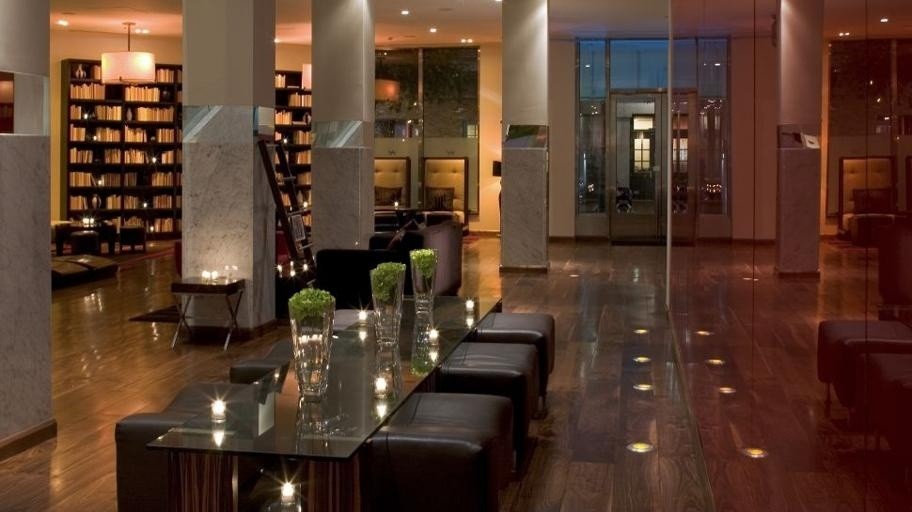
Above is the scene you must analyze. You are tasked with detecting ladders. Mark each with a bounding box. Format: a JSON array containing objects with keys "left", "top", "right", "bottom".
[{"left": 258, "top": 139, "right": 315, "bottom": 272}]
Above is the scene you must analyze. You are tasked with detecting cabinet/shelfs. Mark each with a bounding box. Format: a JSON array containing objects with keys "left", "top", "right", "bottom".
[
  {"left": 60, "top": 58, "right": 183, "bottom": 240},
  {"left": 275, "top": 70, "right": 312, "bottom": 237}
]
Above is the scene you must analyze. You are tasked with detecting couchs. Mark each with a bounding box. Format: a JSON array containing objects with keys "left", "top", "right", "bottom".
[{"left": 316, "top": 219, "right": 465, "bottom": 308}]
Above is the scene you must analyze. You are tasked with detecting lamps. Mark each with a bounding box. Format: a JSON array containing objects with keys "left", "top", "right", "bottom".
[
  {"left": 375, "top": 79, "right": 401, "bottom": 102},
  {"left": 302, "top": 64, "right": 312, "bottom": 92},
  {"left": 101, "top": 22, "right": 156, "bottom": 85}
]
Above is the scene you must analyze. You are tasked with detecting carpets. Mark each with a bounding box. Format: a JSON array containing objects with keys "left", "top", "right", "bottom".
[{"left": 128, "top": 303, "right": 290, "bottom": 326}]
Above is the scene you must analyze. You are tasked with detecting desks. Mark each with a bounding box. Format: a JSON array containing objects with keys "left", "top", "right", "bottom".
[
  {"left": 170, "top": 276, "right": 245, "bottom": 352},
  {"left": 145, "top": 295, "right": 502, "bottom": 512},
  {"left": 55, "top": 222, "right": 117, "bottom": 255}
]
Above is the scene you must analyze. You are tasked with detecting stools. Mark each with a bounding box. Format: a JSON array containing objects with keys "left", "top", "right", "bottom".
[
  {"left": 815, "top": 318, "right": 912, "bottom": 488},
  {"left": 120, "top": 227, "right": 147, "bottom": 253},
  {"left": 70, "top": 230, "right": 100, "bottom": 256}
]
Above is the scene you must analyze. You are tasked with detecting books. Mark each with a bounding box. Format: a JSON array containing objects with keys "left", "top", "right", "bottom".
[
  {"left": 67, "top": 61, "right": 182, "bottom": 234},
  {"left": 274, "top": 72, "right": 312, "bottom": 228}
]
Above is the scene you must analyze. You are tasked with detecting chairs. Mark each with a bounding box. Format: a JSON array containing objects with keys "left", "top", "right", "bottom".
[
  {"left": 839, "top": 155, "right": 898, "bottom": 247},
  {"left": 374, "top": 156, "right": 469, "bottom": 236}
]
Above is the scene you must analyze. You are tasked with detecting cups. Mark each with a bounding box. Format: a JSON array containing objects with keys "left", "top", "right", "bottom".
[
  {"left": 289, "top": 296, "right": 334, "bottom": 396},
  {"left": 370, "top": 268, "right": 405, "bottom": 348},
  {"left": 410, "top": 249, "right": 439, "bottom": 319}
]
